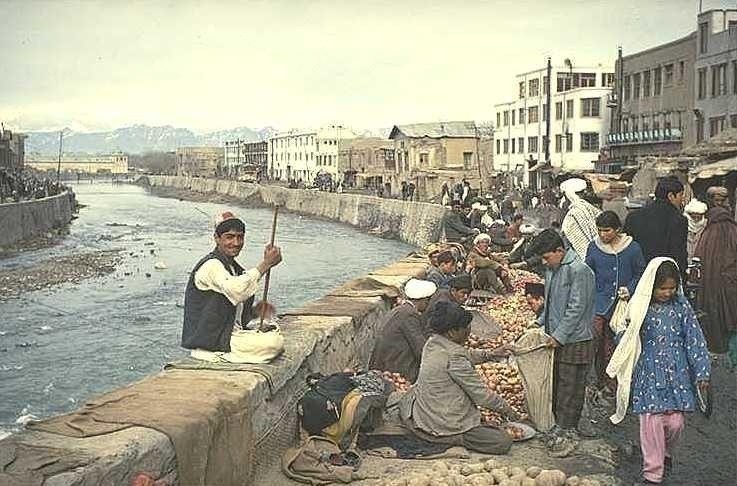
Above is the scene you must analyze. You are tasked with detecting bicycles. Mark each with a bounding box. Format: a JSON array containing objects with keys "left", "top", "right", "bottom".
[{"left": 679, "top": 257, "right": 714, "bottom": 420}]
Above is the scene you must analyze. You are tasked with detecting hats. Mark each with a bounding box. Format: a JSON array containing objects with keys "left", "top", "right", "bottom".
[
  {"left": 472, "top": 201, "right": 537, "bottom": 234},
  {"left": 472, "top": 234, "right": 491, "bottom": 245},
  {"left": 707, "top": 185, "right": 730, "bottom": 200},
  {"left": 212, "top": 207, "right": 241, "bottom": 235},
  {"left": 559, "top": 179, "right": 588, "bottom": 195},
  {"left": 684, "top": 202, "right": 707, "bottom": 214},
  {"left": 403, "top": 277, "right": 437, "bottom": 300}
]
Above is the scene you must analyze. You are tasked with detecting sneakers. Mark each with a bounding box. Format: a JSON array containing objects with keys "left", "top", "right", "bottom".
[{"left": 540, "top": 425, "right": 578, "bottom": 443}]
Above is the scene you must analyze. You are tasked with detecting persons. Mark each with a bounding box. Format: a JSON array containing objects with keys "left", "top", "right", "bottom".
[{"left": 179, "top": 210, "right": 285, "bottom": 364}]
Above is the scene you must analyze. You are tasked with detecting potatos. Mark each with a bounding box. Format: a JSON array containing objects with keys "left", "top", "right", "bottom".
[
  {"left": 342, "top": 247, "right": 560, "bottom": 443},
  {"left": 361, "top": 461, "right": 598, "bottom": 486}
]
